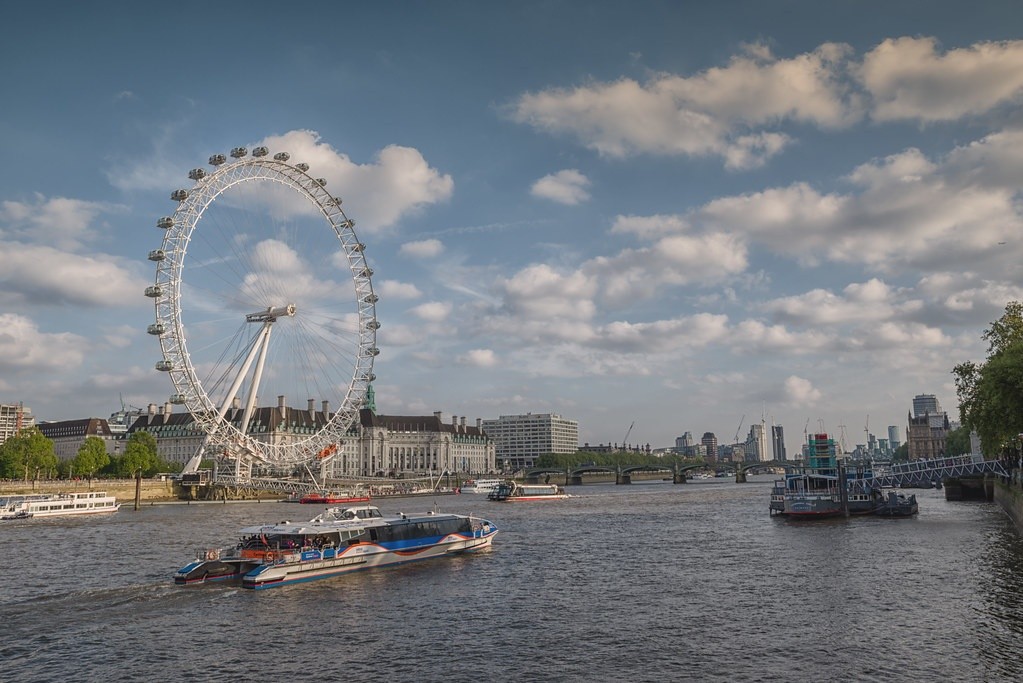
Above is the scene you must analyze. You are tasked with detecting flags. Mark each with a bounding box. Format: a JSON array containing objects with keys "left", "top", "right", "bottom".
[{"left": 260, "top": 532, "right": 267, "bottom": 545}]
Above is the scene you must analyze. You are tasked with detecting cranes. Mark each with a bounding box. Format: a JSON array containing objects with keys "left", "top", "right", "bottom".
[
  {"left": 622, "top": 421, "right": 635, "bottom": 445},
  {"left": 804, "top": 418, "right": 809, "bottom": 442},
  {"left": 864, "top": 414, "right": 869, "bottom": 442},
  {"left": 734, "top": 415, "right": 745, "bottom": 444},
  {"left": 761, "top": 400, "right": 768, "bottom": 424}
]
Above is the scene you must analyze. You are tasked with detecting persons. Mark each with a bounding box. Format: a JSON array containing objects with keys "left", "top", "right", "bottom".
[
  {"left": 239, "top": 534, "right": 268, "bottom": 549},
  {"left": 204, "top": 548, "right": 217, "bottom": 560},
  {"left": 287, "top": 535, "right": 334, "bottom": 552}
]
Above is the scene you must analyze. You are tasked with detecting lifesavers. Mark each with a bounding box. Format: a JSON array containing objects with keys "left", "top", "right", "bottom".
[
  {"left": 209, "top": 550, "right": 217, "bottom": 560},
  {"left": 473, "top": 525, "right": 479, "bottom": 530},
  {"left": 266, "top": 552, "right": 275, "bottom": 560}
]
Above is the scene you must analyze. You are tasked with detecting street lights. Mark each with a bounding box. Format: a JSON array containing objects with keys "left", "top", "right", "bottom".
[
  {"left": 89, "top": 464, "right": 94, "bottom": 489},
  {"left": 32, "top": 465, "right": 38, "bottom": 490}
]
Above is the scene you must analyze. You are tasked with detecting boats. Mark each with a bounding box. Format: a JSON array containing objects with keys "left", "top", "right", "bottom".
[
  {"left": 300, "top": 483, "right": 370, "bottom": 504},
  {"left": 0, "top": 492, "right": 121, "bottom": 519},
  {"left": 173, "top": 506, "right": 499, "bottom": 590},
  {"left": 769, "top": 433, "right": 885, "bottom": 519},
  {"left": 875, "top": 491, "right": 918, "bottom": 516},
  {"left": 506, "top": 484, "right": 572, "bottom": 503},
  {"left": 460, "top": 479, "right": 505, "bottom": 494}
]
